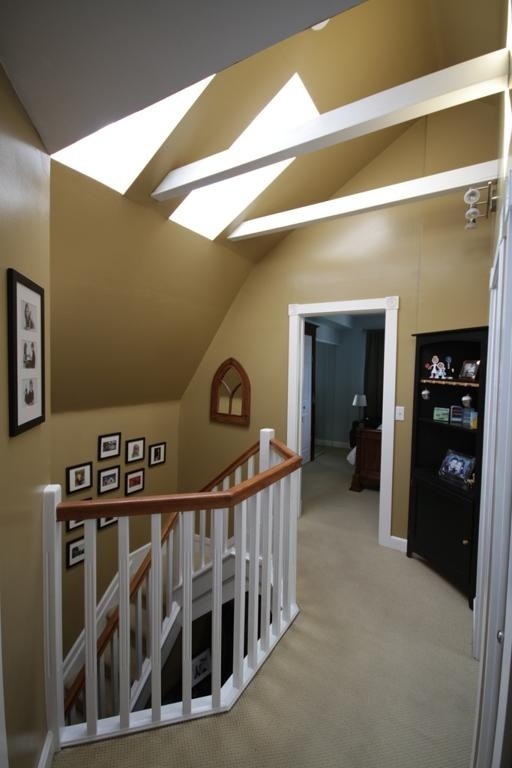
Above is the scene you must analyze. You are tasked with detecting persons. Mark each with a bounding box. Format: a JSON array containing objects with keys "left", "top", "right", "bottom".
[
  {"left": 24, "top": 304, "right": 35, "bottom": 330},
  {"left": 25, "top": 380, "right": 34, "bottom": 404},
  {"left": 446, "top": 457, "right": 465, "bottom": 478},
  {"left": 131, "top": 444, "right": 140, "bottom": 458},
  {"left": 22, "top": 341, "right": 35, "bottom": 368}
]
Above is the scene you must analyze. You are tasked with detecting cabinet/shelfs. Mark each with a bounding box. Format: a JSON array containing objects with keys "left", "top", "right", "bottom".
[{"left": 406, "top": 324, "right": 490, "bottom": 610}]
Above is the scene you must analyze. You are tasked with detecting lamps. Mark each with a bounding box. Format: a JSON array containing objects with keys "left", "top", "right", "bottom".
[
  {"left": 463, "top": 177, "right": 497, "bottom": 232},
  {"left": 352, "top": 393, "right": 368, "bottom": 421}
]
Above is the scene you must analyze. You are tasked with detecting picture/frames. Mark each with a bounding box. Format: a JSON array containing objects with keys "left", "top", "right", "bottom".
[
  {"left": 5, "top": 266, "right": 47, "bottom": 438},
  {"left": 64, "top": 432, "right": 168, "bottom": 569}
]
